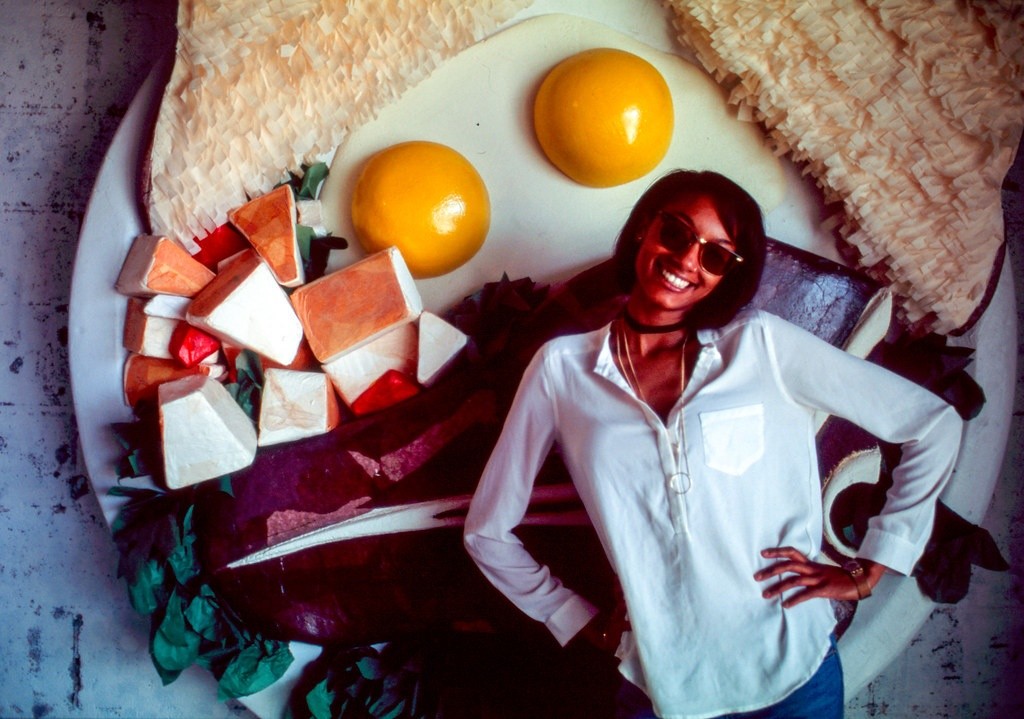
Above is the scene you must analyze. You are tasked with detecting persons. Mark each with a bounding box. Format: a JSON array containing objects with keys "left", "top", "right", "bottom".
[{"left": 463, "top": 171, "right": 964, "bottom": 719}]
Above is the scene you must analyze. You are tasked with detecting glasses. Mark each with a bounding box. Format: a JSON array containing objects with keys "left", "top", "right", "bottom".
[{"left": 653, "top": 209, "right": 745, "bottom": 277}]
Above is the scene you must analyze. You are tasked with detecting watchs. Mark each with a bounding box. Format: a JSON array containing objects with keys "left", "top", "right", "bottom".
[{"left": 843, "top": 561, "right": 872, "bottom": 600}]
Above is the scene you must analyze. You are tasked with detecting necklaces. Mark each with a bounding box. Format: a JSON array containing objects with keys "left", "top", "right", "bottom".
[{"left": 608, "top": 306, "right": 694, "bottom": 493}]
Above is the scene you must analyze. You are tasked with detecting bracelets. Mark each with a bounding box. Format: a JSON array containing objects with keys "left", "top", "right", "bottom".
[{"left": 601, "top": 617, "right": 611, "bottom": 656}]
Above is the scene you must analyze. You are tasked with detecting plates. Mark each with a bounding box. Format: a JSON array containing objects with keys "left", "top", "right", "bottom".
[{"left": 67, "top": 2, "right": 1018, "bottom": 719}]
